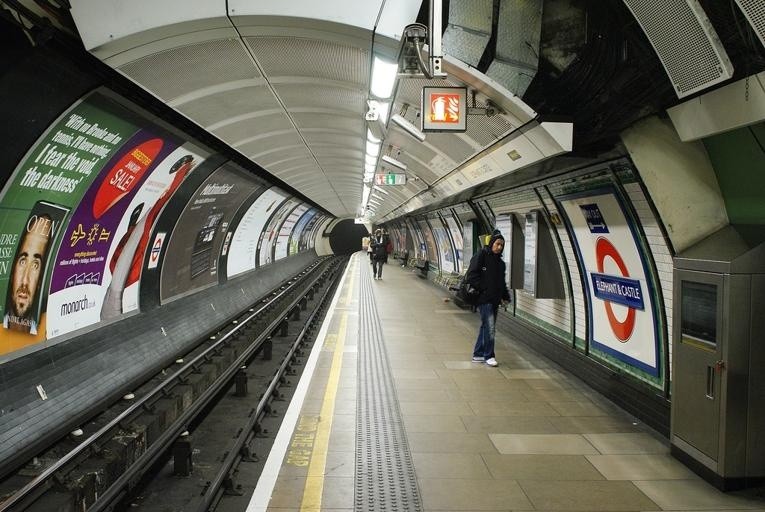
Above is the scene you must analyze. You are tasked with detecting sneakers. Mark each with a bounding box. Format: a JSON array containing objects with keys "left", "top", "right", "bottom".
[
  {"left": 486, "top": 358, "right": 498, "bottom": 366},
  {"left": 472, "top": 357, "right": 484, "bottom": 363}
]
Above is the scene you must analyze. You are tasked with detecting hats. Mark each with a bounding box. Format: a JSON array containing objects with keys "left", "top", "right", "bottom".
[{"left": 489, "top": 230, "right": 504, "bottom": 253}]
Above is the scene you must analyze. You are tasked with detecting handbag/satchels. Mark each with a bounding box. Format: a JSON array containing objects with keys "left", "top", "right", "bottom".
[{"left": 453, "top": 267, "right": 487, "bottom": 310}]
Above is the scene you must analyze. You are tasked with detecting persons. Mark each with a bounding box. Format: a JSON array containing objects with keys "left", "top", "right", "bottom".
[
  {"left": 11, "top": 214, "right": 52, "bottom": 317},
  {"left": 366, "top": 233, "right": 393, "bottom": 264},
  {"left": 460, "top": 229, "right": 512, "bottom": 366},
  {"left": 373, "top": 229, "right": 385, "bottom": 281}
]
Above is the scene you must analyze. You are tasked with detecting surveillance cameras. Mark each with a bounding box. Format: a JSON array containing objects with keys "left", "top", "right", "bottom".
[
  {"left": 366, "top": 108, "right": 388, "bottom": 140},
  {"left": 397, "top": 22, "right": 432, "bottom": 79},
  {"left": 362, "top": 177, "right": 372, "bottom": 188}
]
